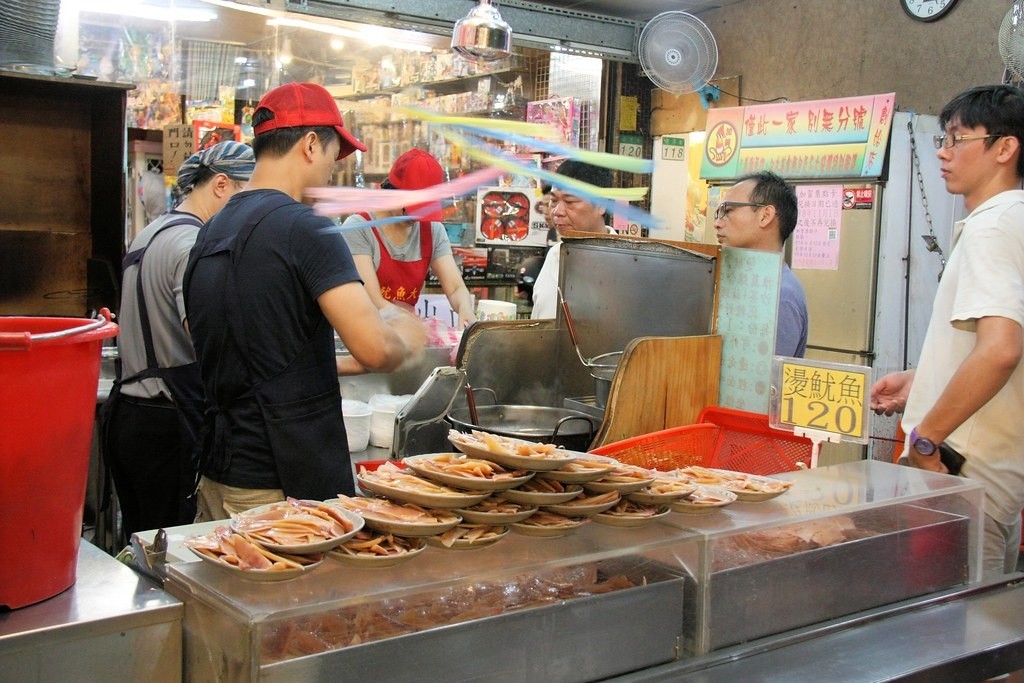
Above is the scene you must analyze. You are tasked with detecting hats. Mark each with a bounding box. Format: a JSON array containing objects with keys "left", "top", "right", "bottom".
[
  {"left": 253, "top": 82, "right": 367, "bottom": 160},
  {"left": 390, "top": 148, "right": 447, "bottom": 222}
]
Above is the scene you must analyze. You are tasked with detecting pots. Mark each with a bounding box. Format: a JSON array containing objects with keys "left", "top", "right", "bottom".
[{"left": 447, "top": 387, "right": 601, "bottom": 452}]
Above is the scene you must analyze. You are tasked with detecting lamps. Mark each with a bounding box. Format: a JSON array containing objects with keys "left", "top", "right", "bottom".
[{"left": 451, "top": 1, "right": 513, "bottom": 62}]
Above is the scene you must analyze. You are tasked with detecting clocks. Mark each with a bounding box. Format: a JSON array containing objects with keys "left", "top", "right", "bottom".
[{"left": 900, "top": 0, "right": 958, "bottom": 22}]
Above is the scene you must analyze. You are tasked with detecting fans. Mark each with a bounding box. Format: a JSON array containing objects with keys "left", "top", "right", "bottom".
[
  {"left": 998, "top": 0, "right": 1024, "bottom": 92},
  {"left": 638, "top": 12, "right": 721, "bottom": 109}
]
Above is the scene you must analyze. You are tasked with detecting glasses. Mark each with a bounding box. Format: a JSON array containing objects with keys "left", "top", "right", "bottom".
[
  {"left": 712, "top": 201, "right": 779, "bottom": 221},
  {"left": 933, "top": 131, "right": 1005, "bottom": 150}
]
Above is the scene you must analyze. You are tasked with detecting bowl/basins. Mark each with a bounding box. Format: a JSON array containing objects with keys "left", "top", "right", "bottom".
[
  {"left": 476, "top": 299, "right": 517, "bottom": 322},
  {"left": 344, "top": 394, "right": 395, "bottom": 453}
]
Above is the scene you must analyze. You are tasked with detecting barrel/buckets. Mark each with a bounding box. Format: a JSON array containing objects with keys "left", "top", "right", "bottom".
[
  {"left": 0, "top": 306, "right": 119, "bottom": 610},
  {"left": 590, "top": 372, "right": 613, "bottom": 409},
  {"left": 442, "top": 222, "right": 467, "bottom": 243}
]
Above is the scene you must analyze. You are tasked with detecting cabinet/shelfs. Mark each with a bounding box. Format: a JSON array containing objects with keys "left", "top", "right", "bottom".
[{"left": 332, "top": 67, "right": 529, "bottom": 185}]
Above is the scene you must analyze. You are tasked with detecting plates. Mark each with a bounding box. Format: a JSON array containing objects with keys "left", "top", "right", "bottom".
[{"left": 187, "top": 433, "right": 790, "bottom": 584}]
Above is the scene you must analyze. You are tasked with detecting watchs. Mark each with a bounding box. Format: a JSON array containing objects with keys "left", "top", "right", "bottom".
[{"left": 907, "top": 426, "right": 941, "bottom": 456}]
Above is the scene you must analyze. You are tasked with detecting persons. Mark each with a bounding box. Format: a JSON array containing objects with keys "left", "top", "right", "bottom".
[
  {"left": 339, "top": 149, "right": 481, "bottom": 349},
  {"left": 714, "top": 172, "right": 809, "bottom": 359},
  {"left": 530, "top": 156, "right": 621, "bottom": 321},
  {"left": 182, "top": 85, "right": 425, "bottom": 525},
  {"left": 870, "top": 85, "right": 1024, "bottom": 583},
  {"left": 113, "top": 140, "right": 257, "bottom": 527}
]
